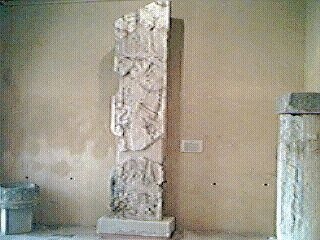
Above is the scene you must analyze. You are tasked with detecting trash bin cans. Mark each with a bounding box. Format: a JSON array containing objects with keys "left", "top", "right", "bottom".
[{"left": 1, "top": 182, "right": 40, "bottom": 235}]
